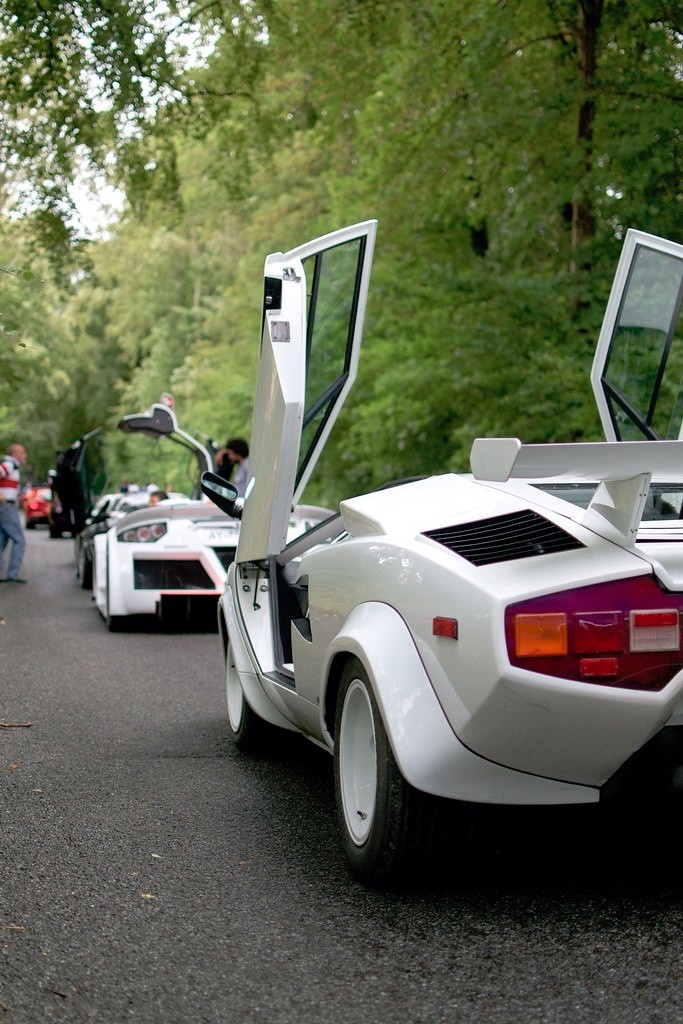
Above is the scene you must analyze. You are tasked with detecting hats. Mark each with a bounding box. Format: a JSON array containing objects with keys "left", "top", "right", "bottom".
[{"left": 226, "top": 439, "right": 249, "bottom": 458}]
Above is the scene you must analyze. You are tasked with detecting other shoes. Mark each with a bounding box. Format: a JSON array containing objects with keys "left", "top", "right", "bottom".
[{"left": 1, "top": 577, "right": 29, "bottom": 584}]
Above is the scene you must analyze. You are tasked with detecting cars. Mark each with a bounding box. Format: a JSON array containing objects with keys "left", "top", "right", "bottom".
[
  {"left": 75, "top": 485, "right": 190, "bottom": 587},
  {"left": 21, "top": 481, "right": 52, "bottom": 529}
]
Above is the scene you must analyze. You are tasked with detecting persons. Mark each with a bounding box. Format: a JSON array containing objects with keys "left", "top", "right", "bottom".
[
  {"left": 0, "top": 443, "right": 28, "bottom": 584},
  {"left": 148, "top": 493, "right": 168, "bottom": 506},
  {"left": 115, "top": 478, "right": 172, "bottom": 493},
  {"left": 214, "top": 438, "right": 249, "bottom": 497}
]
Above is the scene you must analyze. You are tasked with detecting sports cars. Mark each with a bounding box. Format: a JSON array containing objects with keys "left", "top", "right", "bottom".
[
  {"left": 92, "top": 399, "right": 339, "bottom": 632},
  {"left": 216, "top": 218, "right": 681, "bottom": 888}
]
